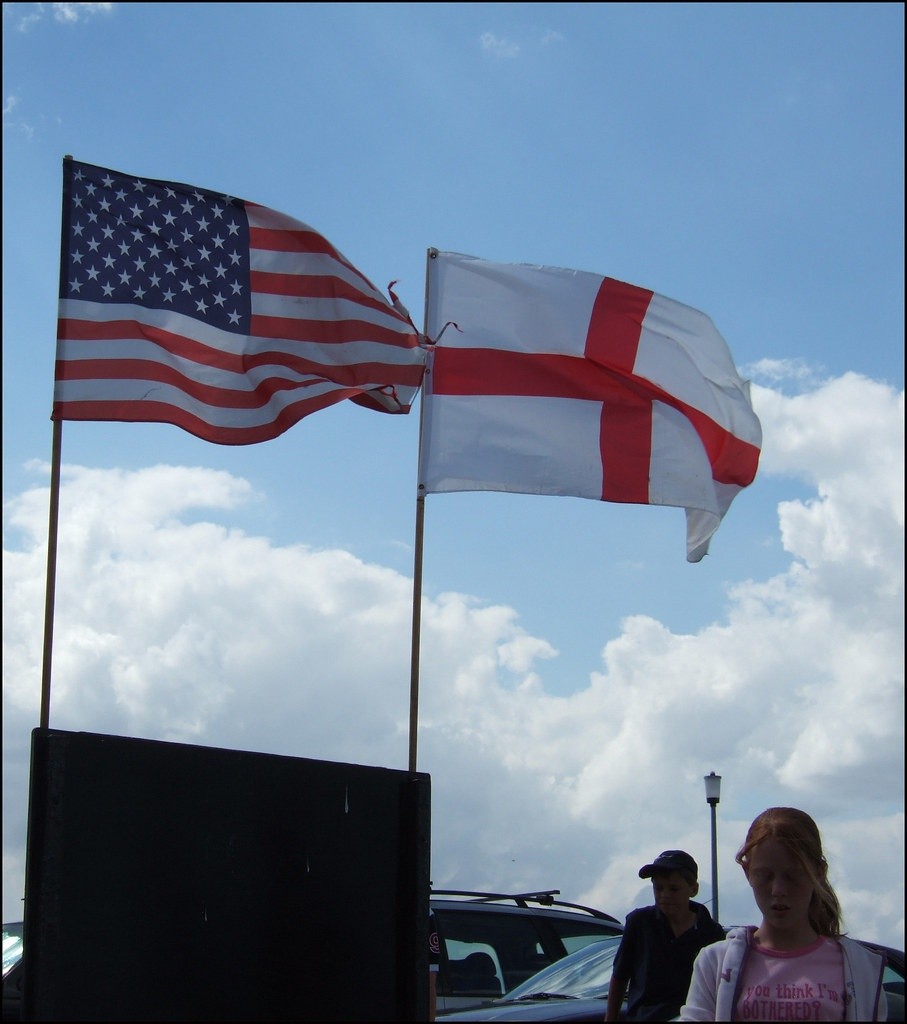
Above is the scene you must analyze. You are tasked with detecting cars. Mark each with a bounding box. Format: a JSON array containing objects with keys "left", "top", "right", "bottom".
[{"left": 432, "top": 884, "right": 906, "bottom": 1024}]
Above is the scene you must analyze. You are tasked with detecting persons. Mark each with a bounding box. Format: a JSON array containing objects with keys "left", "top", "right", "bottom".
[
  {"left": 667, "top": 808, "right": 890, "bottom": 1022},
  {"left": 427, "top": 907, "right": 444, "bottom": 1022},
  {"left": 606, "top": 849, "right": 727, "bottom": 1021}
]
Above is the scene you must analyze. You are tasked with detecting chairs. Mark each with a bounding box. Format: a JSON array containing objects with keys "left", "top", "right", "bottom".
[{"left": 448, "top": 952, "right": 501, "bottom": 990}]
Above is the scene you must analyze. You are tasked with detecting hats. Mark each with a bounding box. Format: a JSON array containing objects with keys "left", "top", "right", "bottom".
[{"left": 639, "top": 851, "right": 698, "bottom": 879}]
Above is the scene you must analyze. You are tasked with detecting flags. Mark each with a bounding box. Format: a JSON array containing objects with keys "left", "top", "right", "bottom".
[
  {"left": 417, "top": 247, "right": 762, "bottom": 564},
  {"left": 52, "top": 157, "right": 433, "bottom": 448}
]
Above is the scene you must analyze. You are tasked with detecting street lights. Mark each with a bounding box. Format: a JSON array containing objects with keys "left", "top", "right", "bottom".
[{"left": 702, "top": 771, "right": 723, "bottom": 923}]
